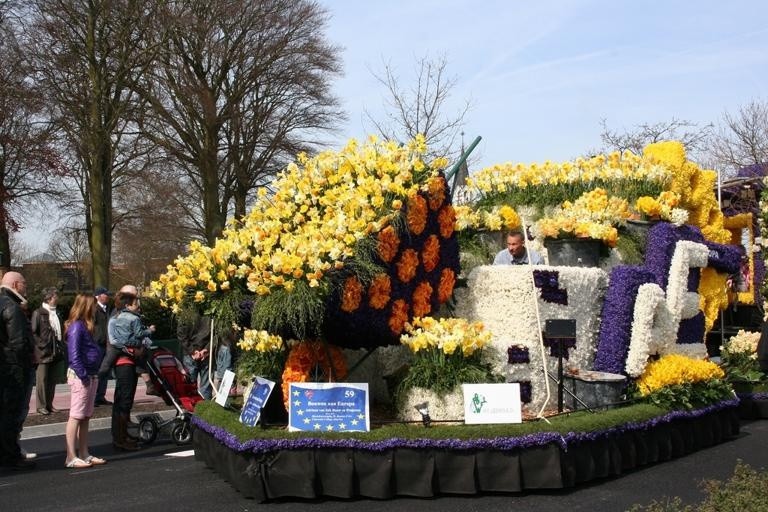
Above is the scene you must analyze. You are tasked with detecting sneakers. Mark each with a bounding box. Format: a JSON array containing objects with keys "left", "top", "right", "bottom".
[
  {"left": 93, "top": 399, "right": 112, "bottom": 407},
  {"left": 126, "top": 422, "right": 140, "bottom": 428},
  {"left": 112, "top": 441, "right": 141, "bottom": 452},
  {"left": 37, "top": 408, "right": 49, "bottom": 414},
  {"left": 125, "top": 435, "right": 145, "bottom": 443},
  {"left": 0, "top": 461, "right": 36, "bottom": 472},
  {"left": 46, "top": 407, "right": 59, "bottom": 413}
]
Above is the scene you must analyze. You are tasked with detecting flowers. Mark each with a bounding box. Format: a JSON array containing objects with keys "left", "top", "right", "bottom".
[
  {"left": 536, "top": 211, "right": 619, "bottom": 246},
  {"left": 453, "top": 204, "right": 521, "bottom": 233}
]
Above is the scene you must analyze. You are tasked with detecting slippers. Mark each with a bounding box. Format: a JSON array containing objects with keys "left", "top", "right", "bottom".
[
  {"left": 65, "top": 457, "right": 92, "bottom": 468},
  {"left": 84, "top": 455, "right": 106, "bottom": 464}
]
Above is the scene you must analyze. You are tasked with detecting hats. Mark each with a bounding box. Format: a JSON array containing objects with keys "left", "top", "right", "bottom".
[{"left": 94, "top": 287, "right": 112, "bottom": 296}]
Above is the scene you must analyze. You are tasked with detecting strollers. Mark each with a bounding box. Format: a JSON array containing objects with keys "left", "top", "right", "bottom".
[{"left": 137, "top": 340, "right": 205, "bottom": 445}]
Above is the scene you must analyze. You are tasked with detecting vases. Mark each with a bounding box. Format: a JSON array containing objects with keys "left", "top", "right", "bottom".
[
  {"left": 477, "top": 229, "right": 505, "bottom": 254},
  {"left": 541, "top": 239, "right": 601, "bottom": 267}
]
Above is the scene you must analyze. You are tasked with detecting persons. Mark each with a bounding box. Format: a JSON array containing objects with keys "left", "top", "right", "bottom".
[
  {"left": 64, "top": 294, "right": 107, "bottom": 468},
  {"left": 107, "top": 285, "right": 144, "bottom": 451},
  {"left": 17, "top": 294, "right": 37, "bottom": 459},
  {"left": 0, "top": 271, "right": 37, "bottom": 471},
  {"left": 217, "top": 344, "right": 237, "bottom": 395},
  {"left": 90, "top": 287, "right": 113, "bottom": 407},
  {"left": 178, "top": 313, "right": 211, "bottom": 401},
  {"left": 31, "top": 286, "right": 65, "bottom": 414},
  {"left": 493, "top": 232, "right": 544, "bottom": 265},
  {"left": 95, "top": 293, "right": 161, "bottom": 396}
]
